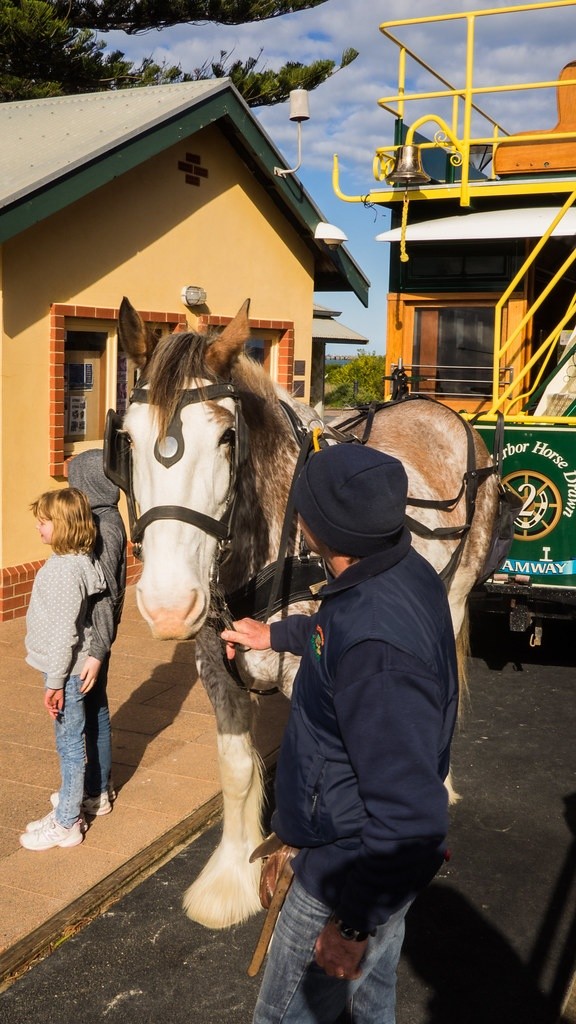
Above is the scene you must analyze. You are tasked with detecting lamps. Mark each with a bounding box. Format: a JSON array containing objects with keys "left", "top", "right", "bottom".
[
  {"left": 181, "top": 285, "right": 208, "bottom": 307},
  {"left": 273, "top": 85, "right": 310, "bottom": 179}
]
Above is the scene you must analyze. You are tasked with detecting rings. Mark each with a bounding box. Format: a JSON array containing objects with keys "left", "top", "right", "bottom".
[{"left": 337, "top": 974, "right": 344, "bottom": 977}]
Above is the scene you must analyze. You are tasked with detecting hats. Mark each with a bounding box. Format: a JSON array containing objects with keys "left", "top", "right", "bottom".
[{"left": 291, "top": 443, "right": 409, "bottom": 558}]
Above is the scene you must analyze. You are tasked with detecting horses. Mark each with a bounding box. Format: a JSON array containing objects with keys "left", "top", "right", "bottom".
[{"left": 113, "top": 292, "right": 518, "bottom": 927}]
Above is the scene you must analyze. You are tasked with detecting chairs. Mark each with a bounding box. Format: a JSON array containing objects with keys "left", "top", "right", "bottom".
[{"left": 494, "top": 60, "right": 576, "bottom": 176}]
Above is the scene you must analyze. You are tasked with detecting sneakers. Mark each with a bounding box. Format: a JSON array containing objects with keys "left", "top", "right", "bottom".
[
  {"left": 50, "top": 781, "right": 116, "bottom": 816},
  {"left": 25, "top": 808, "right": 89, "bottom": 834},
  {"left": 19, "top": 819, "right": 83, "bottom": 851}
]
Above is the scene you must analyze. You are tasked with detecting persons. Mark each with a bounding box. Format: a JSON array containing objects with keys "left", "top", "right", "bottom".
[
  {"left": 51, "top": 450, "right": 128, "bottom": 816},
  {"left": 19, "top": 487, "right": 107, "bottom": 851},
  {"left": 220, "top": 444, "right": 458, "bottom": 1024}
]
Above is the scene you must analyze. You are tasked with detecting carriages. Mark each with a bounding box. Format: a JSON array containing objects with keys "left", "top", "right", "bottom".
[{"left": 102, "top": 0, "right": 576, "bottom": 931}]
